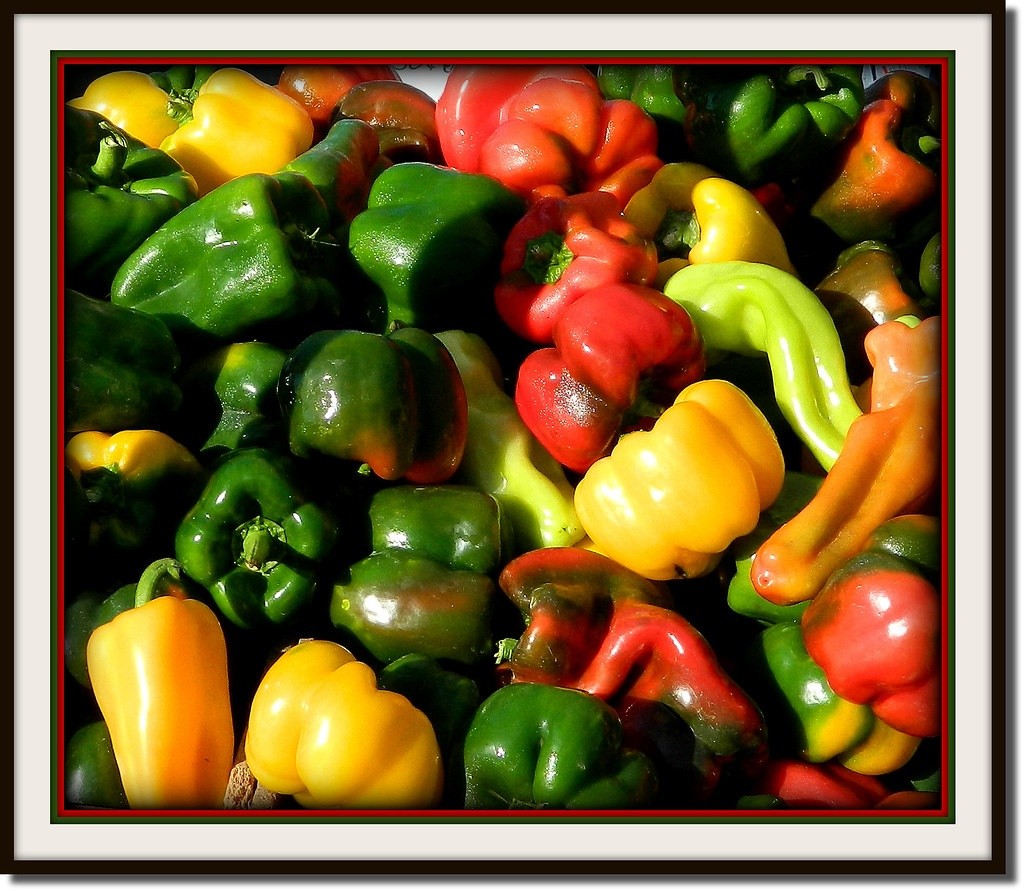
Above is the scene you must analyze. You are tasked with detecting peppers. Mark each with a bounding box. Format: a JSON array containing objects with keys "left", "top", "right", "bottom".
[{"left": 65, "top": 66, "right": 943, "bottom": 809}]
